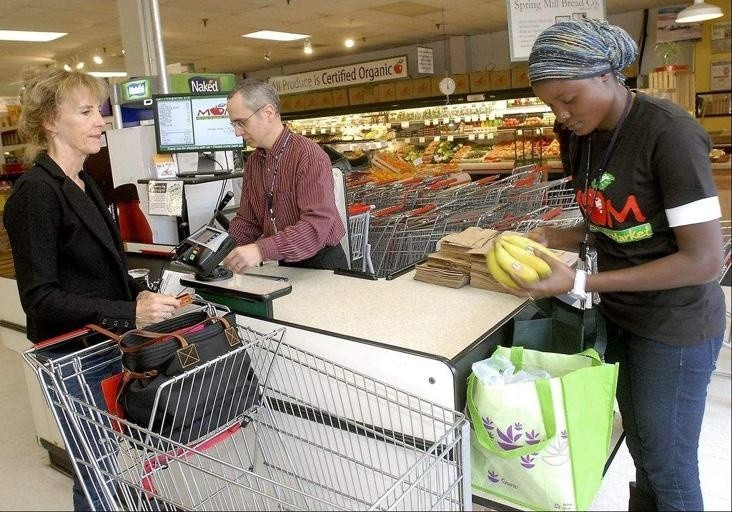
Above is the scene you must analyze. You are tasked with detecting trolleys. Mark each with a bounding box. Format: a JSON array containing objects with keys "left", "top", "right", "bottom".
[
  {"left": 345, "top": 160, "right": 582, "bottom": 280},
  {"left": 16, "top": 294, "right": 475, "bottom": 512},
  {"left": 715, "top": 218, "right": 732, "bottom": 350}
]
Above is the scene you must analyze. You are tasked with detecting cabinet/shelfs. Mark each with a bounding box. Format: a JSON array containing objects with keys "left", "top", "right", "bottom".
[
  {"left": 280, "top": 97, "right": 570, "bottom": 172},
  {"left": 0, "top": 112, "right": 49, "bottom": 327}
]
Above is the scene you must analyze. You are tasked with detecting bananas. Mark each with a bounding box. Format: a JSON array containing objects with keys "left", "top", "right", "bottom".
[{"left": 487, "top": 234, "right": 560, "bottom": 291}]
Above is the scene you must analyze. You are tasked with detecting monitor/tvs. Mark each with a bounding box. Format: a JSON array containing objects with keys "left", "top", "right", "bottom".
[{"left": 152, "top": 91, "right": 246, "bottom": 177}]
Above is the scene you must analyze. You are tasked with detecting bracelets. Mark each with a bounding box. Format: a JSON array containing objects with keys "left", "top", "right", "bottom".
[{"left": 566, "top": 266, "right": 596, "bottom": 313}]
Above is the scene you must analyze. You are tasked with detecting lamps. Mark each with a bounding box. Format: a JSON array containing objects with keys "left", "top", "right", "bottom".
[
  {"left": 304, "top": 39, "right": 314, "bottom": 54},
  {"left": 675, "top": 0, "right": 724, "bottom": 23},
  {"left": 264, "top": 50, "right": 272, "bottom": 61}
]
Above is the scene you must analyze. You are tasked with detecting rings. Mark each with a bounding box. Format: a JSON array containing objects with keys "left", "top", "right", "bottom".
[
  {"left": 164, "top": 313, "right": 172, "bottom": 319},
  {"left": 525, "top": 290, "right": 537, "bottom": 303}
]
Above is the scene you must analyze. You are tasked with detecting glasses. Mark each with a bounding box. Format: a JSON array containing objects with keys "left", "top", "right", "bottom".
[{"left": 228, "top": 107, "right": 263, "bottom": 130}]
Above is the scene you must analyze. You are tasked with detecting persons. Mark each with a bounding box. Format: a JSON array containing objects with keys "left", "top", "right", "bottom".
[
  {"left": 501, "top": 16, "right": 729, "bottom": 512},
  {"left": 218, "top": 74, "right": 353, "bottom": 277},
  {"left": 0, "top": 61, "right": 182, "bottom": 512}
]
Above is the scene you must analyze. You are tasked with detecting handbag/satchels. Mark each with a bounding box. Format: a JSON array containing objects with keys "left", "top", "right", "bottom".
[
  {"left": 462, "top": 345, "right": 620, "bottom": 512},
  {"left": 118, "top": 311, "right": 259, "bottom": 454}
]
